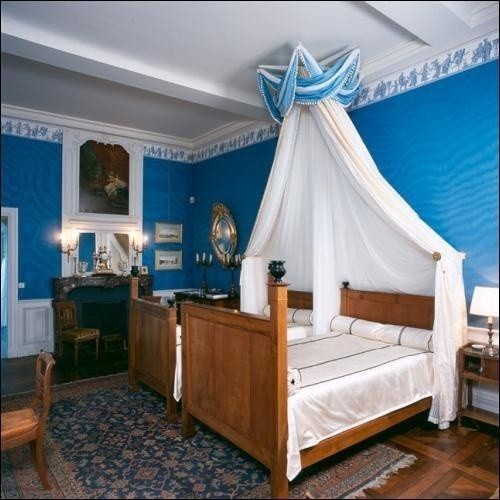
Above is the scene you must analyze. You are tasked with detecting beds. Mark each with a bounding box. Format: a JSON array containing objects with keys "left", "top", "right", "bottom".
[
  {"left": 181, "top": 260, "right": 453, "bottom": 500},
  {"left": 126, "top": 264, "right": 313, "bottom": 427}
]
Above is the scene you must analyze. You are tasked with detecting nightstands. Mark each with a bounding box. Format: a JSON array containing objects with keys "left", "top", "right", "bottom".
[{"left": 456, "top": 341, "right": 500, "bottom": 434}]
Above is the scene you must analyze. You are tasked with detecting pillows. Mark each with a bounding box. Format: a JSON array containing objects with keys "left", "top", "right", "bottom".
[
  {"left": 330, "top": 314, "right": 434, "bottom": 351},
  {"left": 264, "top": 304, "right": 313, "bottom": 324}
]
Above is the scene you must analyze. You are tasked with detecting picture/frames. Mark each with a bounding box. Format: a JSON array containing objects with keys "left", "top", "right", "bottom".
[
  {"left": 155, "top": 222, "right": 183, "bottom": 244},
  {"left": 155, "top": 249, "right": 182, "bottom": 271},
  {"left": 68, "top": 131, "right": 138, "bottom": 225},
  {"left": 209, "top": 202, "right": 237, "bottom": 264}
]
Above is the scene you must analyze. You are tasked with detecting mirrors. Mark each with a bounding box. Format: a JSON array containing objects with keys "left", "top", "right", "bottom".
[{"left": 76, "top": 230, "right": 134, "bottom": 276}]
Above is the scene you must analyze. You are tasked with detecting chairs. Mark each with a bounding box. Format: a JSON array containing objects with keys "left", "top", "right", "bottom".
[
  {"left": 0, "top": 350, "right": 58, "bottom": 491},
  {"left": 53, "top": 299, "right": 103, "bottom": 368}
]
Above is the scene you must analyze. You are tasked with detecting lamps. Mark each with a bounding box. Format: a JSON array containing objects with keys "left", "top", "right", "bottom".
[{"left": 469, "top": 285, "right": 499, "bottom": 353}]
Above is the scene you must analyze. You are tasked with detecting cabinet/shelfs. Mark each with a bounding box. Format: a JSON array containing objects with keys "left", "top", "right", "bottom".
[{"left": 173, "top": 289, "right": 239, "bottom": 326}]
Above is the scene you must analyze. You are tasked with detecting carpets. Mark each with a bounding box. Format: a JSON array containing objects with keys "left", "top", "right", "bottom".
[{"left": 0, "top": 371, "right": 422, "bottom": 499}]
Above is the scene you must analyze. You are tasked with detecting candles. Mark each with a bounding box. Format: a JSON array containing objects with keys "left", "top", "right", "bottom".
[{"left": 195, "top": 252, "right": 241, "bottom": 265}]
